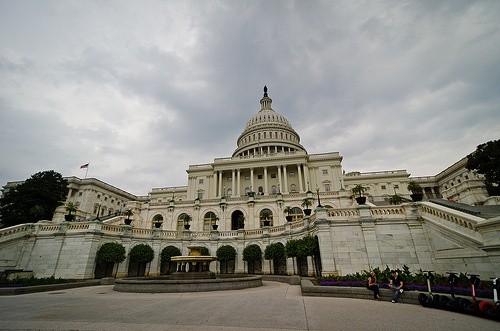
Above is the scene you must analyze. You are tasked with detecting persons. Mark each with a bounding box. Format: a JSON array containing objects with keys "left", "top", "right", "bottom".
[
  {"left": 389, "top": 270, "right": 403, "bottom": 302},
  {"left": 366, "top": 271, "right": 381, "bottom": 300}
]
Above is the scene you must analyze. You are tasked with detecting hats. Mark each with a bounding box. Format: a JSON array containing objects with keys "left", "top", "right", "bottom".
[{"left": 390, "top": 270, "right": 396, "bottom": 273}]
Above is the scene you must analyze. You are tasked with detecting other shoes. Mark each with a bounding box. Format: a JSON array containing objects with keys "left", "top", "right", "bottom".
[
  {"left": 391, "top": 299, "right": 395, "bottom": 303},
  {"left": 399, "top": 289, "right": 403, "bottom": 294},
  {"left": 377, "top": 294, "right": 381, "bottom": 299}
]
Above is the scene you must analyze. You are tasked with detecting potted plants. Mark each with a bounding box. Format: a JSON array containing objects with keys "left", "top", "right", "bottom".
[
  {"left": 237, "top": 215, "right": 245, "bottom": 228},
  {"left": 211, "top": 214, "right": 220, "bottom": 230},
  {"left": 155, "top": 214, "right": 163, "bottom": 228},
  {"left": 184, "top": 214, "right": 193, "bottom": 229},
  {"left": 352, "top": 184, "right": 366, "bottom": 204},
  {"left": 122, "top": 210, "right": 133, "bottom": 225},
  {"left": 407, "top": 180, "right": 423, "bottom": 201},
  {"left": 261, "top": 212, "right": 270, "bottom": 226},
  {"left": 284, "top": 206, "right": 293, "bottom": 222},
  {"left": 29, "top": 205, "right": 44, "bottom": 223},
  {"left": 63, "top": 202, "right": 79, "bottom": 221},
  {"left": 300, "top": 198, "right": 312, "bottom": 215}
]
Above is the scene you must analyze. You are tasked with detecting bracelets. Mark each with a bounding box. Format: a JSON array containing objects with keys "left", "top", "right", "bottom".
[{"left": 390, "top": 283, "right": 392, "bottom": 285}]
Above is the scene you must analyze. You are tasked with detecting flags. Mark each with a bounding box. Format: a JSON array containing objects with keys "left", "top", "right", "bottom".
[{"left": 81, "top": 163, "right": 89, "bottom": 168}]
